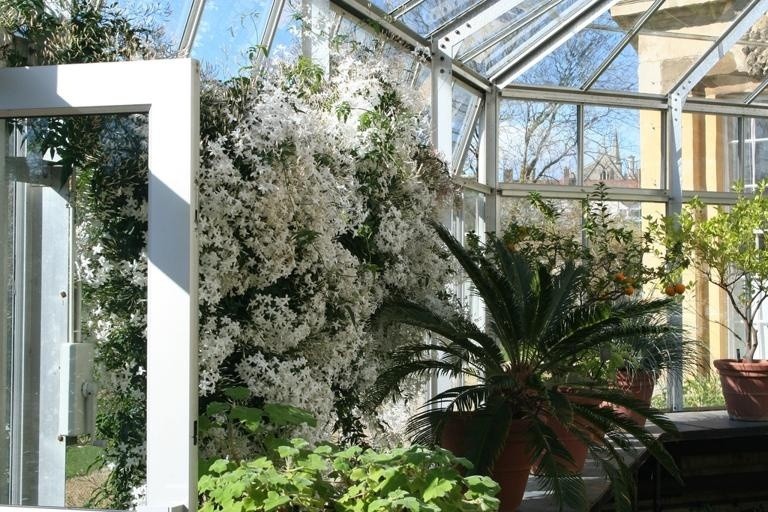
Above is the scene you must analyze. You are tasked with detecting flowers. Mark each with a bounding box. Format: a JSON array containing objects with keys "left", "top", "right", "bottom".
[{"left": 2, "top": 3, "right": 468, "bottom": 512}]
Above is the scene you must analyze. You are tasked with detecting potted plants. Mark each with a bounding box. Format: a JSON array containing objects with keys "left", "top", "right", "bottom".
[
  {"left": 507, "top": 203, "right": 609, "bottom": 477},
  {"left": 647, "top": 181, "right": 767, "bottom": 421},
  {"left": 585, "top": 186, "right": 690, "bottom": 428},
  {"left": 372, "top": 214, "right": 679, "bottom": 510}
]
